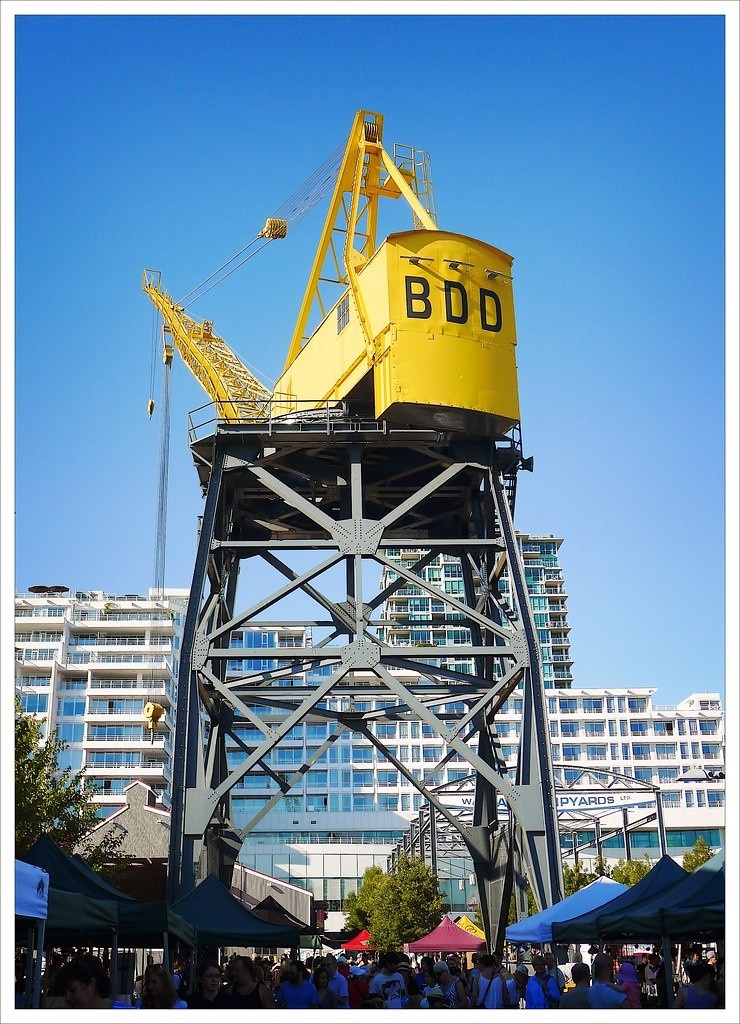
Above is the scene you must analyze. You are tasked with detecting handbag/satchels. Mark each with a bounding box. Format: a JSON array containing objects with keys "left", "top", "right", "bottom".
[
  {"left": 550, "top": 993, "right": 564, "bottom": 1009},
  {"left": 470, "top": 1002, "right": 485, "bottom": 1009}
]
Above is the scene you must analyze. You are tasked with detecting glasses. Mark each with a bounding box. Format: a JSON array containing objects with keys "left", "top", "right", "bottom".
[
  {"left": 435, "top": 970, "right": 444, "bottom": 977},
  {"left": 322, "top": 963, "right": 334, "bottom": 968}
]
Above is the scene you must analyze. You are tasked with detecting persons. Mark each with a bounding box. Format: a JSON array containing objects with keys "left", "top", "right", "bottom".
[
  {"left": 526, "top": 956, "right": 562, "bottom": 1009},
  {"left": 275, "top": 960, "right": 319, "bottom": 1009},
  {"left": 135, "top": 963, "right": 188, "bottom": 1009},
  {"left": 16, "top": 937, "right": 724, "bottom": 1009},
  {"left": 470, "top": 954, "right": 511, "bottom": 1010}
]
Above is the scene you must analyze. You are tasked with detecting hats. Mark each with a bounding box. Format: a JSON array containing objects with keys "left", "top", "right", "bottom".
[
  {"left": 429, "top": 988, "right": 444, "bottom": 999},
  {"left": 347, "top": 964, "right": 366, "bottom": 978},
  {"left": 616, "top": 963, "right": 637, "bottom": 983},
  {"left": 398, "top": 962, "right": 412, "bottom": 971},
  {"left": 705, "top": 951, "right": 715, "bottom": 961},
  {"left": 518, "top": 965, "right": 528, "bottom": 974},
  {"left": 478, "top": 955, "right": 496, "bottom": 966}
]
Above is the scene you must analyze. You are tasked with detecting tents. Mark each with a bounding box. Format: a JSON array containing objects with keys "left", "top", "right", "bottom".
[
  {"left": 551, "top": 849, "right": 725, "bottom": 941},
  {"left": 16, "top": 831, "right": 322, "bottom": 948},
  {"left": 505, "top": 875, "right": 632, "bottom": 944},
  {"left": 342, "top": 925, "right": 381, "bottom": 951},
  {"left": 409, "top": 916, "right": 488, "bottom": 953}
]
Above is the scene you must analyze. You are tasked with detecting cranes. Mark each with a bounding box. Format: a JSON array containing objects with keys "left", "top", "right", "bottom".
[{"left": 141, "top": 111, "right": 570, "bottom": 965}]
